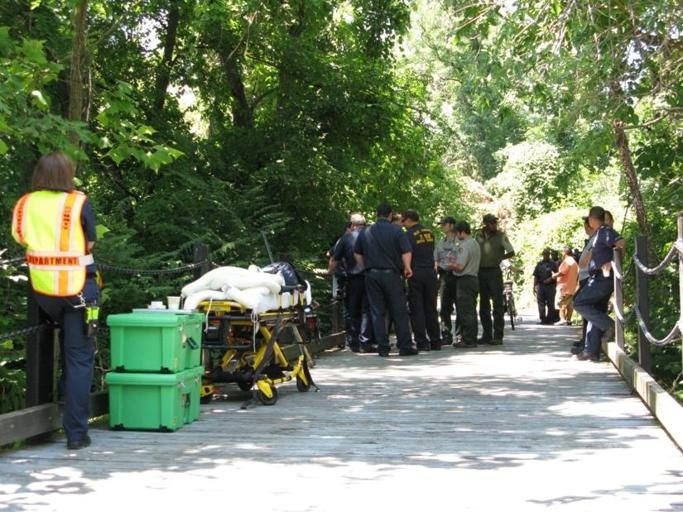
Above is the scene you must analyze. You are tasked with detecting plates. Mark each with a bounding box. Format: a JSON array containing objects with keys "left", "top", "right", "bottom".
[{"left": 147, "top": 305, "right": 166, "bottom": 311}]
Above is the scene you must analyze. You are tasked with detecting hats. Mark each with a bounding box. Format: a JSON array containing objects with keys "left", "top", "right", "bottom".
[
  {"left": 484, "top": 214, "right": 498, "bottom": 224},
  {"left": 436, "top": 218, "right": 456, "bottom": 225}
]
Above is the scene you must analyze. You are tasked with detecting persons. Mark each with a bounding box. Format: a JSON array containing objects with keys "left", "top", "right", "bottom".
[
  {"left": 12, "top": 150, "right": 103, "bottom": 449},
  {"left": 325, "top": 198, "right": 514, "bottom": 357},
  {"left": 531, "top": 205, "right": 626, "bottom": 359}
]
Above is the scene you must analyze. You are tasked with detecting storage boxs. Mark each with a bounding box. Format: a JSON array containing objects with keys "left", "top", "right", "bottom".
[
  {"left": 106, "top": 311, "right": 205, "bottom": 373},
  {"left": 104, "top": 367, "right": 206, "bottom": 433}
]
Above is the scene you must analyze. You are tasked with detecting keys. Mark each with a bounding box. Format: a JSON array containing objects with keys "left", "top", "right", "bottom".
[{"left": 73, "top": 292, "right": 87, "bottom": 309}]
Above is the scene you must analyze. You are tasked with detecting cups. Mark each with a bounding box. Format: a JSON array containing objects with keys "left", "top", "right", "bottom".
[
  {"left": 166, "top": 296, "right": 180, "bottom": 310},
  {"left": 150, "top": 301, "right": 163, "bottom": 307}
]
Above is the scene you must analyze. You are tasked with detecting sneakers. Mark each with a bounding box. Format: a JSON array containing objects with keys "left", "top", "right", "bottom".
[
  {"left": 601, "top": 326, "right": 614, "bottom": 342},
  {"left": 68, "top": 436, "right": 90, "bottom": 449},
  {"left": 454, "top": 337, "right": 502, "bottom": 348},
  {"left": 571, "top": 341, "right": 600, "bottom": 360},
  {"left": 350, "top": 338, "right": 452, "bottom": 355}
]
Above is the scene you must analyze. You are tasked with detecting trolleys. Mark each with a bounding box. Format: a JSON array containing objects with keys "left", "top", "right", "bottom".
[{"left": 194, "top": 299, "right": 320, "bottom": 407}]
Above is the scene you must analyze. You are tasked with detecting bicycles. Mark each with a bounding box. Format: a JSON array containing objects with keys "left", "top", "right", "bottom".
[{"left": 499, "top": 268, "right": 528, "bottom": 330}]
